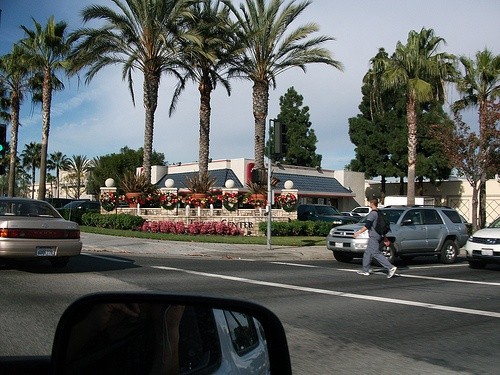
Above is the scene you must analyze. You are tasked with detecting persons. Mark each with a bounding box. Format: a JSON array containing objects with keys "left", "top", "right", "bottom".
[
  {"left": 354, "top": 198, "right": 397, "bottom": 279},
  {"left": 67, "top": 303, "right": 186, "bottom": 375}
]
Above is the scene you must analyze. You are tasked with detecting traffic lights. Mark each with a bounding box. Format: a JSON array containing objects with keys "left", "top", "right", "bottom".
[{"left": 274, "top": 120, "right": 291, "bottom": 154}]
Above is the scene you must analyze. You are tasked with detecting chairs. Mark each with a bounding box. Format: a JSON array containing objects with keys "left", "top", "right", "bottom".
[{"left": 20, "top": 202, "right": 38, "bottom": 217}]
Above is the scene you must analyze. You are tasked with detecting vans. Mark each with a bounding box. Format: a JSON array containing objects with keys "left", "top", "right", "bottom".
[{"left": 351, "top": 206, "right": 384, "bottom": 217}]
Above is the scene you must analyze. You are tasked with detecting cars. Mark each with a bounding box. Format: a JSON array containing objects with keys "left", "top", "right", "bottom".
[
  {"left": 0, "top": 197, "right": 83, "bottom": 266},
  {"left": 464, "top": 216, "right": 500, "bottom": 268},
  {"left": 117, "top": 202, "right": 130, "bottom": 208},
  {"left": 341, "top": 211, "right": 360, "bottom": 217}
]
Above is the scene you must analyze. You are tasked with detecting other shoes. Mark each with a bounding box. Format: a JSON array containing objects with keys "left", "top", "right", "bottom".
[
  {"left": 357, "top": 271, "right": 370, "bottom": 275},
  {"left": 386, "top": 267, "right": 397, "bottom": 279}
]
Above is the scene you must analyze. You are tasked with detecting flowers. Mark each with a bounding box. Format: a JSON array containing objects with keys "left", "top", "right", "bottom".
[{"left": 99, "top": 191, "right": 297, "bottom": 213}]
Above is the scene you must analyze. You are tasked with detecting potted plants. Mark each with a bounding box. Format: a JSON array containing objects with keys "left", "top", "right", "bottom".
[
  {"left": 122, "top": 171, "right": 151, "bottom": 208},
  {"left": 248, "top": 169, "right": 280, "bottom": 209},
  {"left": 184, "top": 169, "right": 216, "bottom": 209}
]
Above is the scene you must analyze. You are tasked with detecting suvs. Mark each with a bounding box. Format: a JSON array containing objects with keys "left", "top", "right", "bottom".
[
  {"left": 325, "top": 204, "right": 469, "bottom": 266},
  {"left": 297, "top": 204, "right": 355, "bottom": 227},
  {"left": 56, "top": 200, "right": 101, "bottom": 223}
]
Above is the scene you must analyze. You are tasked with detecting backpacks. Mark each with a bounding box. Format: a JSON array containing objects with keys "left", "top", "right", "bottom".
[{"left": 372, "top": 210, "right": 391, "bottom": 235}]
